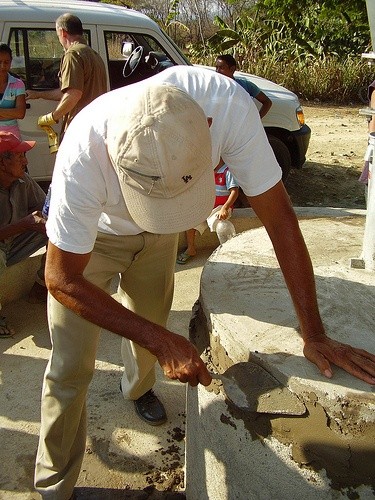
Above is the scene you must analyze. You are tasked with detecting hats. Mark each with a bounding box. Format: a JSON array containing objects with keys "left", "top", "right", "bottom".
[
  {"left": 105, "top": 81, "right": 216, "bottom": 235},
  {"left": 0, "top": 130, "right": 36, "bottom": 156}
]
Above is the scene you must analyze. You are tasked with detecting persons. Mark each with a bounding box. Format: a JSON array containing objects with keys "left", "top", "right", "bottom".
[
  {"left": 215, "top": 54, "right": 273, "bottom": 119},
  {"left": 177, "top": 156, "right": 240, "bottom": 265},
  {"left": 0, "top": 130, "right": 51, "bottom": 338},
  {"left": 358, "top": 79, "right": 375, "bottom": 206},
  {"left": 0, "top": 44, "right": 30, "bottom": 175},
  {"left": 34, "top": 65, "right": 375, "bottom": 500},
  {"left": 37, "top": 12, "right": 108, "bottom": 160}
]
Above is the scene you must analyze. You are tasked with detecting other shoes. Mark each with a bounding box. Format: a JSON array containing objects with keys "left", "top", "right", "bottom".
[
  {"left": 0, "top": 316, "right": 16, "bottom": 338},
  {"left": 28, "top": 295, "right": 47, "bottom": 304}
]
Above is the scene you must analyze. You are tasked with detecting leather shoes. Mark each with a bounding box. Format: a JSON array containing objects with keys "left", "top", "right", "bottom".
[{"left": 119, "top": 378, "right": 167, "bottom": 426}]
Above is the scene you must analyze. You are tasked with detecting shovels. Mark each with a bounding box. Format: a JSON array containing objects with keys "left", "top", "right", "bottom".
[{"left": 205, "top": 360, "right": 308, "bottom": 420}]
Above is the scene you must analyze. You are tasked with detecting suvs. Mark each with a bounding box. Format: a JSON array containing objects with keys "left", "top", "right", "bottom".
[{"left": 0, "top": 0, "right": 312, "bottom": 209}]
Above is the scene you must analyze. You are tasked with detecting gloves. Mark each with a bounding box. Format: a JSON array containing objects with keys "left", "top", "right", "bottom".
[
  {"left": 42, "top": 125, "right": 59, "bottom": 155},
  {"left": 37, "top": 111, "right": 59, "bottom": 130}
]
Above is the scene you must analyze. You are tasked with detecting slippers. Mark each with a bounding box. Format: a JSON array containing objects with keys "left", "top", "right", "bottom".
[{"left": 176, "top": 252, "right": 192, "bottom": 264}]
